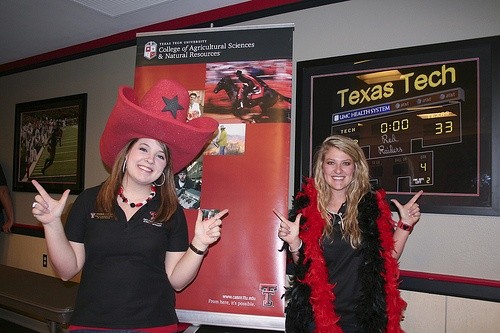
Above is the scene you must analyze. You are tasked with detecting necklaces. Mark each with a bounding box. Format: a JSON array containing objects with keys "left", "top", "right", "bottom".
[{"left": 119, "top": 185, "right": 155, "bottom": 209}]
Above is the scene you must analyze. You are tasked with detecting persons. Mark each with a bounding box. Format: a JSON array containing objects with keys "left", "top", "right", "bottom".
[
  {"left": 235, "top": 68, "right": 262, "bottom": 109},
  {"left": 187, "top": 93, "right": 204, "bottom": 120},
  {"left": 215, "top": 125, "right": 227, "bottom": 155},
  {"left": 21, "top": 119, "right": 49, "bottom": 179},
  {"left": 41, "top": 127, "right": 63, "bottom": 174},
  {"left": 274, "top": 135, "right": 423, "bottom": 333},
  {"left": 32, "top": 138, "right": 230, "bottom": 333},
  {"left": 0, "top": 164, "right": 15, "bottom": 234},
  {"left": 39, "top": 115, "right": 79, "bottom": 129},
  {"left": 173, "top": 167, "right": 194, "bottom": 189}
]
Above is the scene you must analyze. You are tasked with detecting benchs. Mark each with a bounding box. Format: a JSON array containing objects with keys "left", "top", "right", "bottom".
[{"left": 0, "top": 264, "right": 79, "bottom": 333}]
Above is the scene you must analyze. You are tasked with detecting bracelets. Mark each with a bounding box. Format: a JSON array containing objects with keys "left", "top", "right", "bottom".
[
  {"left": 398, "top": 220, "right": 413, "bottom": 231},
  {"left": 289, "top": 238, "right": 303, "bottom": 252},
  {"left": 190, "top": 242, "right": 209, "bottom": 254}
]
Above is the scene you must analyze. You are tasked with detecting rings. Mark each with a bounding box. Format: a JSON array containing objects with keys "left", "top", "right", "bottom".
[{"left": 32, "top": 202, "right": 37, "bottom": 207}]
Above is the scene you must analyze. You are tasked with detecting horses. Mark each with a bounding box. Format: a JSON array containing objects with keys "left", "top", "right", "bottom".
[{"left": 212, "top": 73, "right": 292, "bottom": 124}]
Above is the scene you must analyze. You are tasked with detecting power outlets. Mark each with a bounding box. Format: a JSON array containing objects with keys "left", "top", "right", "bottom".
[{"left": 43, "top": 254, "right": 47, "bottom": 267}]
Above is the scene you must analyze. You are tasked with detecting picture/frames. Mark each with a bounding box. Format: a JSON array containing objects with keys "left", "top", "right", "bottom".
[{"left": 12, "top": 92, "right": 87, "bottom": 195}]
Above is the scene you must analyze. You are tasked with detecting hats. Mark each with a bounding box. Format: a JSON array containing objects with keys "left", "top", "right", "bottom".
[{"left": 100, "top": 80, "right": 219, "bottom": 174}]
